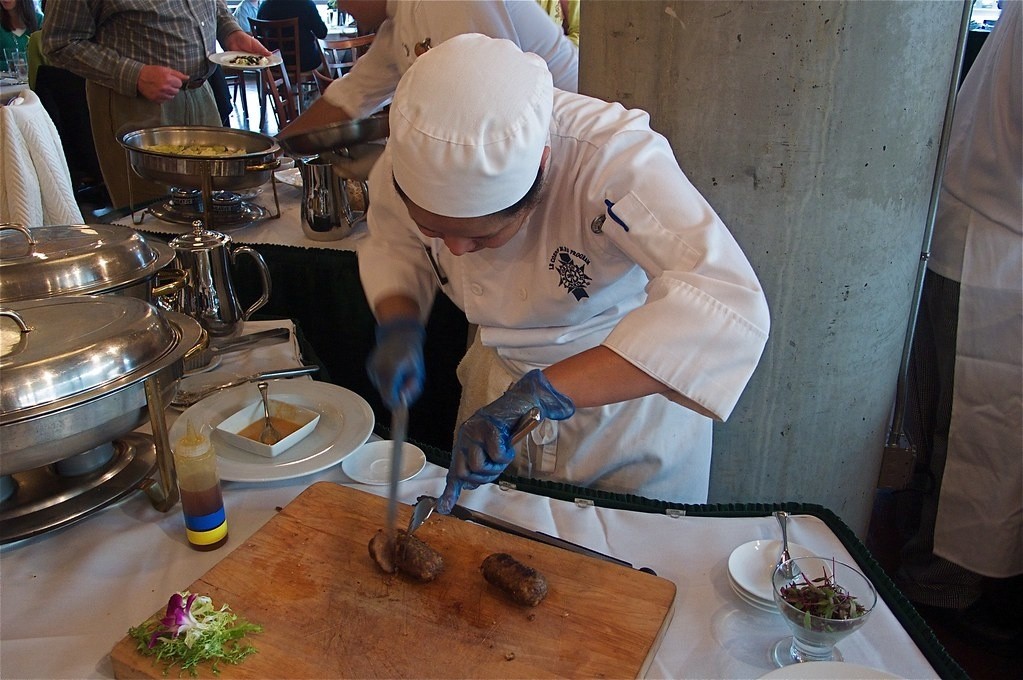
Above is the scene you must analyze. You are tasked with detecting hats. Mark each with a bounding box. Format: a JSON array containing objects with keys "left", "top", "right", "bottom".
[{"left": 388, "top": 31, "right": 554, "bottom": 219}]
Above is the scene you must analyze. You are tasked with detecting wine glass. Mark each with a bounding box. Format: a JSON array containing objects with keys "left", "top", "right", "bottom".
[{"left": 771, "top": 555, "right": 877, "bottom": 670}]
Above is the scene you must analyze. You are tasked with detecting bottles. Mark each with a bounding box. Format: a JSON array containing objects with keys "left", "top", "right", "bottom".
[{"left": 173, "top": 420, "right": 229, "bottom": 551}]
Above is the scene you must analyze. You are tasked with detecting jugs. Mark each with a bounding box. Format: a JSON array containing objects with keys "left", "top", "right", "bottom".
[{"left": 292, "top": 153, "right": 371, "bottom": 243}]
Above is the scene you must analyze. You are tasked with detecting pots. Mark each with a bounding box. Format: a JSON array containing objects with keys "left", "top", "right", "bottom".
[{"left": 0, "top": 122, "right": 286, "bottom": 554}]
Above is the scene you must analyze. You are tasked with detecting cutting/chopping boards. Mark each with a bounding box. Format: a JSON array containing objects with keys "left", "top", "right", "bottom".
[{"left": 108, "top": 475, "right": 679, "bottom": 680}]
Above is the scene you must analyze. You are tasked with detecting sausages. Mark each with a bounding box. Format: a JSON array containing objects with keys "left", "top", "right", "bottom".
[
  {"left": 480, "top": 553, "right": 548, "bottom": 606},
  {"left": 368, "top": 528, "right": 446, "bottom": 582}
]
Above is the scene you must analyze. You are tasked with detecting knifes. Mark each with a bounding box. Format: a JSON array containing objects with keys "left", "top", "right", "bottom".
[{"left": 383, "top": 386, "right": 412, "bottom": 562}]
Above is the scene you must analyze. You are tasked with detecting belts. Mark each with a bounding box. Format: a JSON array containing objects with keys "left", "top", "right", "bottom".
[{"left": 183, "top": 78, "right": 205, "bottom": 90}]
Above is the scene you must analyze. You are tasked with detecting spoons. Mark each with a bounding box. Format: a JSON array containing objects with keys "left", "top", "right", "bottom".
[
  {"left": 257, "top": 382, "right": 281, "bottom": 447},
  {"left": 774, "top": 511, "right": 801, "bottom": 581}
]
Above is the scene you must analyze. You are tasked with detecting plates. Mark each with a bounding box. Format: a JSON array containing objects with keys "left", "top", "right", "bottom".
[
  {"left": 727, "top": 537, "right": 834, "bottom": 613},
  {"left": 167, "top": 379, "right": 375, "bottom": 481},
  {"left": 755, "top": 660, "right": 909, "bottom": 680},
  {"left": 216, "top": 397, "right": 321, "bottom": 458},
  {"left": 208, "top": 50, "right": 284, "bottom": 71},
  {"left": 342, "top": 440, "right": 428, "bottom": 485}
]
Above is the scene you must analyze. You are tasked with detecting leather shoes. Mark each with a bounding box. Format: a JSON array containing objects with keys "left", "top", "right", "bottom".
[{"left": 909, "top": 597, "right": 1023, "bottom": 655}]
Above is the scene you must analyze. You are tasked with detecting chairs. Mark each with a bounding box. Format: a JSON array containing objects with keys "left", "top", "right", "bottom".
[
  {"left": 264, "top": 48, "right": 298, "bottom": 130},
  {"left": 311, "top": 33, "right": 376, "bottom": 80},
  {"left": 224, "top": 69, "right": 263, "bottom": 118},
  {"left": 248, "top": 17, "right": 320, "bottom": 115},
  {"left": 310, "top": 65, "right": 336, "bottom": 97},
  {"left": 35, "top": 65, "right": 115, "bottom": 205}
]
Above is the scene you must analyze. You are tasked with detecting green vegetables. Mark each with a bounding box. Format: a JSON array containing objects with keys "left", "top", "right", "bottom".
[
  {"left": 126, "top": 591, "right": 264, "bottom": 679},
  {"left": 778, "top": 557, "right": 871, "bottom": 635}
]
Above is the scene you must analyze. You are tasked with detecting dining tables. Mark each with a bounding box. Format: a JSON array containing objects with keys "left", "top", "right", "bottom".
[
  {"left": 324, "top": 22, "right": 359, "bottom": 39},
  {"left": 0, "top": 71, "right": 39, "bottom": 103}
]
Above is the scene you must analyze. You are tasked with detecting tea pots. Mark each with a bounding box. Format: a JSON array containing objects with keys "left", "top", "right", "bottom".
[{"left": 167, "top": 222, "right": 270, "bottom": 348}]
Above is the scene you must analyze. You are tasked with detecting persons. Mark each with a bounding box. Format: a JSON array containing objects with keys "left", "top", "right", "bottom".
[
  {"left": 877, "top": 0, "right": 1023, "bottom": 654},
  {"left": 0, "top": 0, "right": 579, "bottom": 211},
  {"left": 358, "top": 33, "right": 771, "bottom": 505}
]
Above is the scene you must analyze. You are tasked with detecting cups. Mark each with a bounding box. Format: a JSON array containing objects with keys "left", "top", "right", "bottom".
[{"left": 3, "top": 47, "right": 28, "bottom": 84}]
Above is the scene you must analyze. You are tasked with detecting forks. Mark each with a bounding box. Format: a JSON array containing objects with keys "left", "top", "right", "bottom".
[
  {"left": 171, "top": 365, "right": 319, "bottom": 411},
  {"left": 403, "top": 410, "right": 542, "bottom": 561}
]
